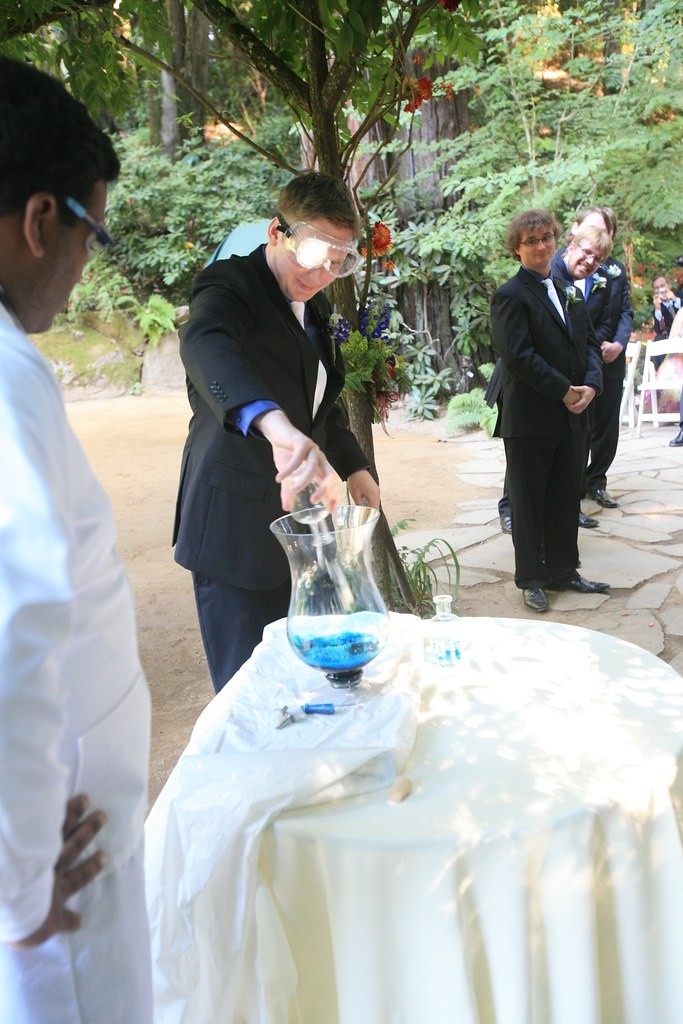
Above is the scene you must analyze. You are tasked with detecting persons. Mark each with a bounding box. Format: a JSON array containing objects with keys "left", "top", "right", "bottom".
[
  {"left": 0, "top": 53, "right": 154, "bottom": 1022},
  {"left": 489, "top": 205, "right": 683, "bottom": 613},
  {"left": 173, "top": 169, "right": 380, "bottom": 699}
]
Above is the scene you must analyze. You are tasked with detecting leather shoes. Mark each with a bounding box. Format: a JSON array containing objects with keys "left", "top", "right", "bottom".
[
  {"left": 586, "top": 490, "right": 618, "bottom": 508},
  {"left": 669, "top": 426, "right": 683, "bottom": 447},
  {"left": 524, "top": 583, "right": 549, "bottom": 612},
  {"left": 500, "top": 515, "right": 513, "bottom": 534},
  {"left": 578, "top": 511, "right": 599, "bottom": 528},
  {"left": 545, "top": 569, "right": 610, "bottom": 593}
]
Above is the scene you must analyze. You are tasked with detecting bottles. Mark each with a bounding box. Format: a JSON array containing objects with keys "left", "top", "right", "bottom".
[
  {"left": 270, "top": 504, "right": 391, "bottom": 706},
  {"left": 427, "top": 594, "right": 465, "bottom": 670}
]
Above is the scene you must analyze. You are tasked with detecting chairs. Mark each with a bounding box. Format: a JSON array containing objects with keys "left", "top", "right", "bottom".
[
  {"left": 636, "top": 337, "right": 683, "bottom": 427},
  {"left": 618, "top": 340, "right": 642, "bottom": 429}
]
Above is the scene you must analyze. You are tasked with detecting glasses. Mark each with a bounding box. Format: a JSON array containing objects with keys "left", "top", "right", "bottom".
[
  {"left": 64, "top": 192, "right": 115, "bottom": 263},
  {"left": 276, "top": 213, "right": 366, "bottom": 280},
  {"left": 578, "top": 244, "right": 603, "bottom": 267},
  {"left": 519, "top": 235, "right": 557, "bottom": 247}
]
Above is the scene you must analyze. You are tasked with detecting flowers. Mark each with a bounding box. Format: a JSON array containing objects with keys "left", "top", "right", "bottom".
[
  {"left": 591, "top": 272, "right": 607, "bottom": 293},
  {"left": 607, "top": 264, "right": 622, "bottom": 280},
  {"left": 326, "top": 302, "right": 347, "bottom": 367},
  {"left": 324, "top": 51, "right": 456, "bottom": 439},
  {"left": 563, "top": 285, "right": 582, "bottom": 313}
]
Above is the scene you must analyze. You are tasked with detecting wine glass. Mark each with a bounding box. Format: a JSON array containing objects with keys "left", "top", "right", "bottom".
[{"left": 289, "top": 478, "right": 335, "bottom": 547}]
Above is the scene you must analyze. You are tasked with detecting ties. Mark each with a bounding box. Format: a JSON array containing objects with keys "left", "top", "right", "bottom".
[
  {"left": 667, "top": 301, "right": 676, "bottom": 320},
  {"left": 574, "top": 279, "right": 585, "bottom": 297},
  {"left": 289, "top": 302, "right": 327, "bottom": 421},
  {"left": 541, "top": 278, "right": 567, "bottom": 325}
]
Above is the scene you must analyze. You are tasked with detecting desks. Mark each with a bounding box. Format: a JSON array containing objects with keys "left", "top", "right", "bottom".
[{"left": 143, "top": 611, "right": 683, "bottom": 1024}]
